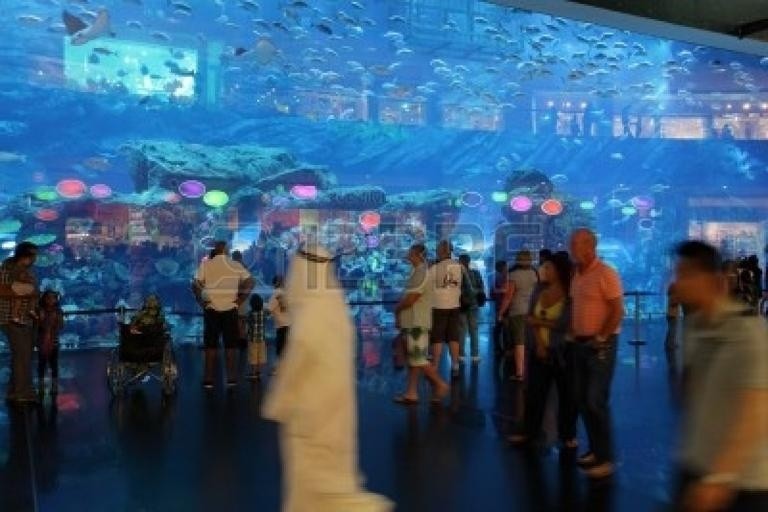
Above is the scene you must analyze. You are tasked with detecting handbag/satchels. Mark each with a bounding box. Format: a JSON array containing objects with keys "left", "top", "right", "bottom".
[
  {"left": 391, "top": 334, "right": 406, "bottom": 371},
  {"left": 476, "top": 291, "right": 487, "bottom": 307}
]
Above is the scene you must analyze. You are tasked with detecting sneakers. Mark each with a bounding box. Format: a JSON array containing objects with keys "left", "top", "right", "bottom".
[
  {"left": 204, "top": 373, "right": 259, "bottom": 389},
  {"left": 578, "top": 453, "right": 617, "bottom": 475},
  {"left": 12, "top": 310, "right": 39, "bottom": 325},
  {"left": 451, "top": 355, "right": 480, "bottom": 372}
]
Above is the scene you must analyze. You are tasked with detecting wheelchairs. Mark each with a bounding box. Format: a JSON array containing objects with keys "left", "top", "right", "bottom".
[{"left": 106, "top": 319, "right": 178, "bottom": 400}]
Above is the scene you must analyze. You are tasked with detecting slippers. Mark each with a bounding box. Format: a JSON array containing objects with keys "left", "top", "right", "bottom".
[{"left": 393, "top": 395, "right": 420, "bottom": 407}]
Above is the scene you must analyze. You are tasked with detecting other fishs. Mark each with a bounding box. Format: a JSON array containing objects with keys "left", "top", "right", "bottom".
[{"left": 1, "top": 0, "right": 767, "bottom": 349}]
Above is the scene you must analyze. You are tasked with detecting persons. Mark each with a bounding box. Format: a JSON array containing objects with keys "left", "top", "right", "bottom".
[
  {"left": 0, "top": 242, "right": 38, "bottom": 404},
  {"left": 393, "top": 227, "right": 625, "bottom": 479},
  {"left": 261, "top": 241, "right": 398, "bottom": 512},
  {"left": 192, "top": 237, "right": 290, "bottom": 390},
  {"left": 667, "top": 241, "right": 768, "bottom": 512},
  {"left": 124, "top": 292, "right": 167, "bottom": 382},
  {"left": 9, "top": 251, "right": 39, "bottom": 326},
  {"left": 35, "top": 289, "right": 64, "bottom": 398}
]
